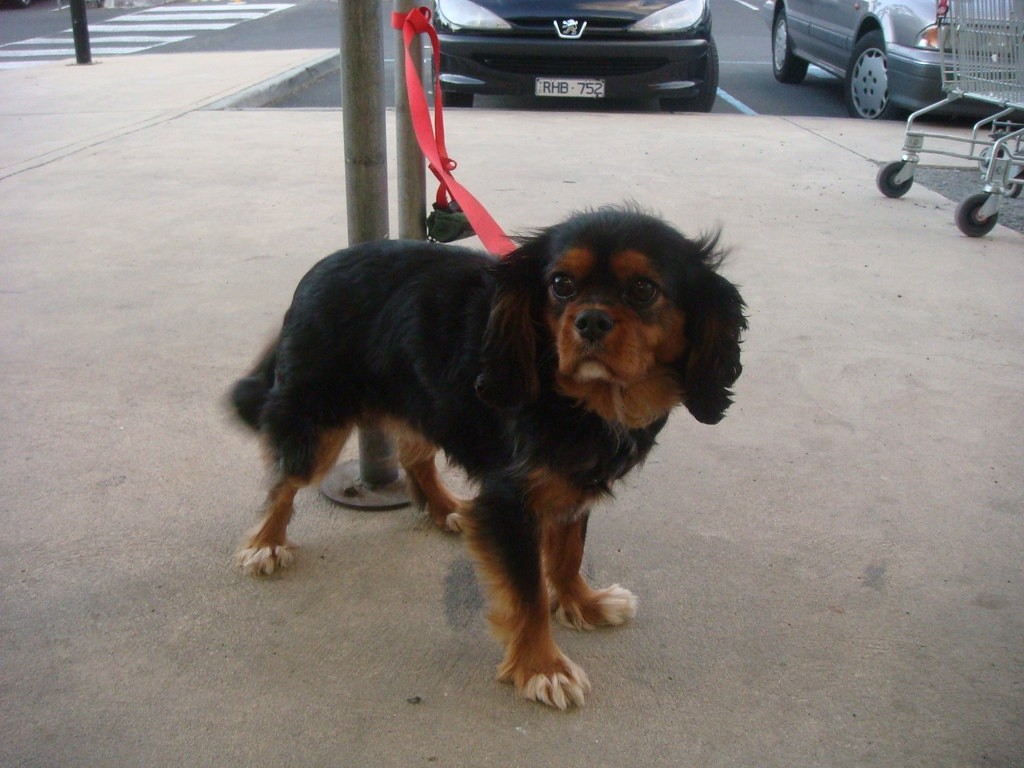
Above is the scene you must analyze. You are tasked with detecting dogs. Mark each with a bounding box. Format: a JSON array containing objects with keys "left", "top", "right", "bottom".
[{"left": 225, "top": 208, "right": 745, "bottom": 713}]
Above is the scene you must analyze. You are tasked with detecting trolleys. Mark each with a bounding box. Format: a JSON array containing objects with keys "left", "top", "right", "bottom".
[{"left": 874, "top": 1, "right": 1024, "bottom": 237}]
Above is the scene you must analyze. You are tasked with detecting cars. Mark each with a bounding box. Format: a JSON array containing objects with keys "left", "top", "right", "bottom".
[
  {"left": 430, "top": 0, "right": 718, "bottom": 112},
  {"left": 762, "top": 1, "right": 1023, "bottom": 120}
]
